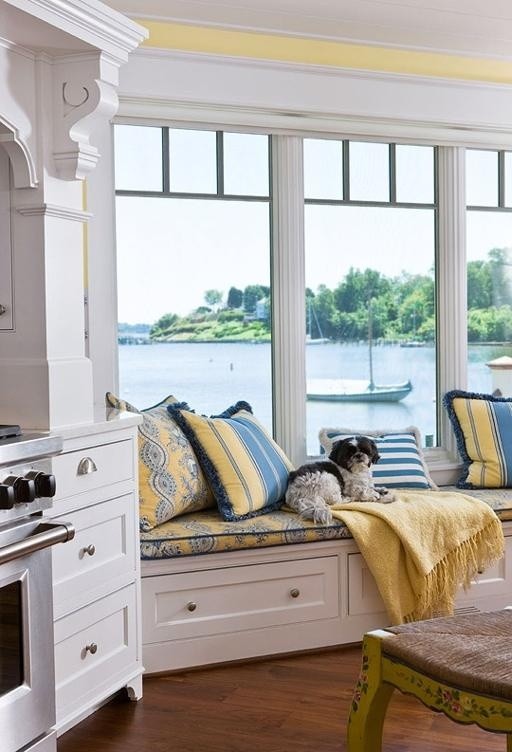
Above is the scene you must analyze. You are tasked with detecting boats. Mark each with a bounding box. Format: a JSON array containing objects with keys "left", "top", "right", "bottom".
[
  {"left": 306, "top": 300, "right": 330, "bottom": 345},
  {"left": 400, "top": 340, "right": 426, "bottom": 347},
  {"left": 486, "top": 356, "right": 512, "bottom": 367},
  {"left": 306, "top": 293, "right": 414, "bottom": 402}
]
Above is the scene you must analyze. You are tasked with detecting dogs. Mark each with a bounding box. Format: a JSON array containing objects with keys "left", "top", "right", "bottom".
[{"left": 284, "top": 436, "right": 396, "bottom": 525}]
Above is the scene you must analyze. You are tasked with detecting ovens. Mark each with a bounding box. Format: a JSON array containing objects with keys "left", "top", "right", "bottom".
[{"left": 0, "top": 458, "right": 76, "bottom": 752}]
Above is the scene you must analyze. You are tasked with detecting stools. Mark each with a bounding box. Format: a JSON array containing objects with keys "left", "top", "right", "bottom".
[{"left": 341, "top": 603, "right": 510, "bottom": 752}]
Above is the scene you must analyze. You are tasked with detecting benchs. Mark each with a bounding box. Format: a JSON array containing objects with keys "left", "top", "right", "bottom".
[{"left": 137, "top": 486, "right": 512, "bottom": 679}]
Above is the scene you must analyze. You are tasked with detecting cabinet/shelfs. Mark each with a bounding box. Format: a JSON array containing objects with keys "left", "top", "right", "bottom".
[{"left": 50, "top": 426, "right": 147, "bottom": 737}]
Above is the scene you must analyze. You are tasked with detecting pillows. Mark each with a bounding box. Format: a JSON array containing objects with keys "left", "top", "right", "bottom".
[
  {"left": 105, "top": 392, "right": 296, "bottom": 533},
  {"left": 318, "top": 425, "right": 440, "bottom": 493},
  {"left": 444, "top": 390, "right": 512, "bottom": 488}
]
